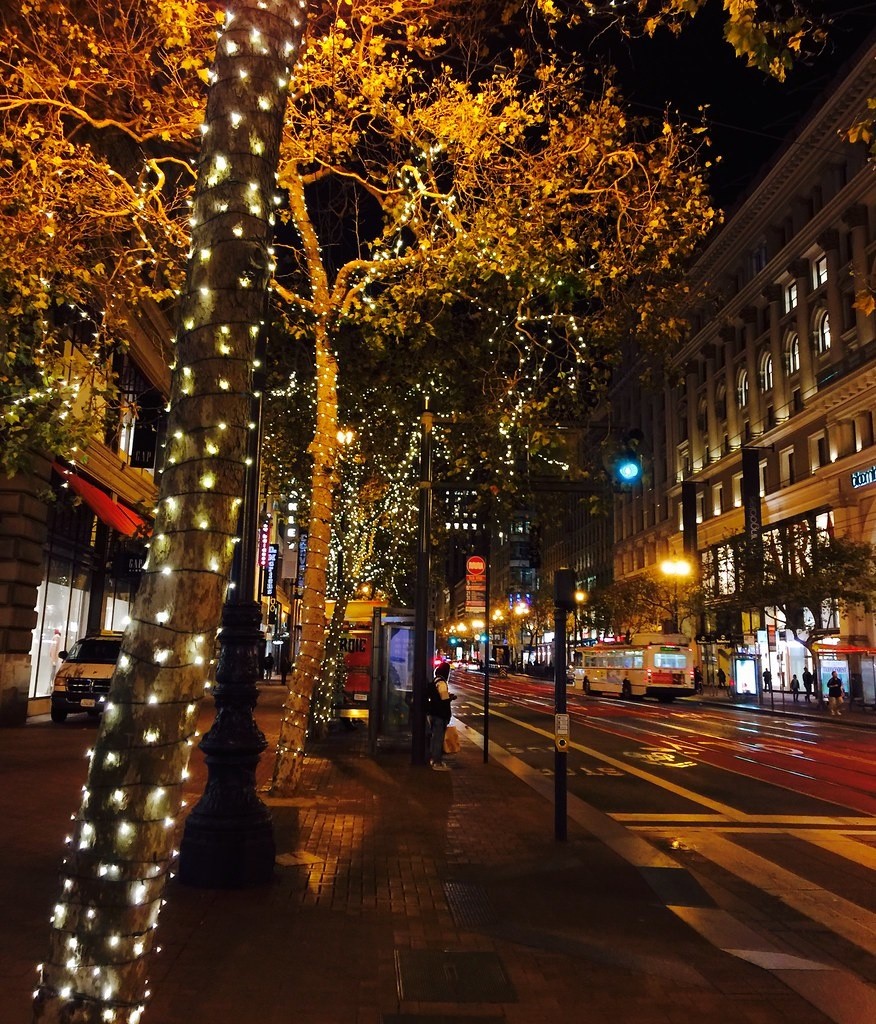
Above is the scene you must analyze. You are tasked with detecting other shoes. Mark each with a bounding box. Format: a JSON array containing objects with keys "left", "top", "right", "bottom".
[
  {"left": 837, "top": 711, "right": 841, "bottom": 716},
  {"left": 429, "top": 758, "right": 452, "bottom": 772},
  {"left": 830, "top": 711, "right": 835, "bottom": 716}
]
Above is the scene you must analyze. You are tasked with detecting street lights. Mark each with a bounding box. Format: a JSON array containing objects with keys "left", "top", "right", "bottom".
[
  {"left": 515, "top": 603, "right": 529, "bottom": 674},
  {"left": 662, "top": 561, "right": 692, "bottom": 623}
]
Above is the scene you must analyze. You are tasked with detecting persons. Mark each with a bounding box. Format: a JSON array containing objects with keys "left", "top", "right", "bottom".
[
  {"left": 280, "top": 656, "right": 289, "bottom": 685},
  {"left": 45, "top": 629, "right": 61, "bottom": 694},
  {"left": 717, "top": 668, "right": 726, "bottom": 689},
  {"left": 429, "top": 662, "right": 457, "bottom": 772},
  {"left": 827, "top": 671, "right": 844, "bottom": 716},
  {"left": 694, "top": 666, "right": 704, "bottom": 694},
  {"left": 802, "top": 667, "right": 813, "bottom": 703},
  {"left": 763, "top": 668, "right": 771, "bottom": 693},
  {"left": 790, "top": 674, "right": 799, "bottom": 703},
  {"left": 724, "top": 673, "right": 733, "bottom": 698},
  {"left": 265, "top": 653, "right": 274, "bottom": 686}
]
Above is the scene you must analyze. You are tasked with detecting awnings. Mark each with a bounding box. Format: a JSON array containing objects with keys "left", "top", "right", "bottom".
[{"left": 55, "top": 466, "right": 155, "bottom": 547}]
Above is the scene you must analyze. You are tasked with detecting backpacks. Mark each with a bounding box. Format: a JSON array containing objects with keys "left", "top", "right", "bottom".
[{"left": 422, "top": 676, "right": 445, "bottom": 716}]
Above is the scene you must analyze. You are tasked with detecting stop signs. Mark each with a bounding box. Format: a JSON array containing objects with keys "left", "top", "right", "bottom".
[{"left": 466, "top": 556, "right": 487, "bottom": 575}]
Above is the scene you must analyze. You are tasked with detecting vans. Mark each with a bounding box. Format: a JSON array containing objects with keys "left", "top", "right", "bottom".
[{"left": 50, "top": 629, "right": 124, "bottom": 724}]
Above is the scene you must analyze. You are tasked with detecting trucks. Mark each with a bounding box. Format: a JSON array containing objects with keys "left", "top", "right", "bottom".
[{"left": 478, "top": 643, "right": 511, "bottom": 674}]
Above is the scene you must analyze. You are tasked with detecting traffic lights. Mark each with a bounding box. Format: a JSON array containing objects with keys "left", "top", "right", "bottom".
[{"left": 620, "top": 423, "right": 645, "bottom": 484}]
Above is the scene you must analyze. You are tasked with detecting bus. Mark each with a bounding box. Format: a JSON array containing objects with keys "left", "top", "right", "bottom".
[{"left": 572, "top": 634, "right": 695, "bottom": 703}]
